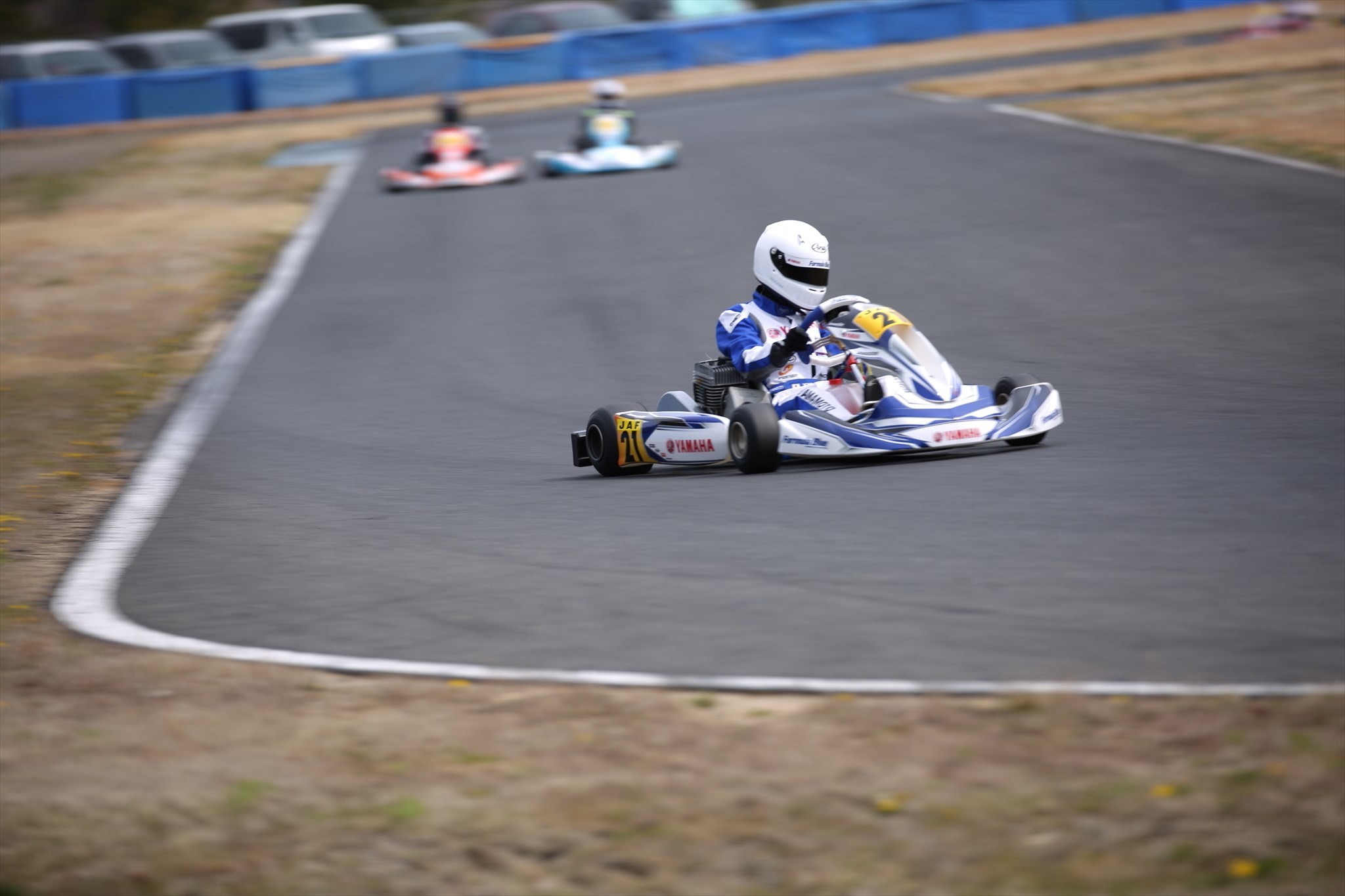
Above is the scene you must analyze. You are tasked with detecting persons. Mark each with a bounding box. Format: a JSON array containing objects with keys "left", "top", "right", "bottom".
[
  {"left": 714, "top": 218, "right": 874, "bottom": 417},
  {"left": 421, "top": 97, "right": 488, "bottom": 170},
  {"left": 577, "top": 80, "right": 642, "bottom": 151}
]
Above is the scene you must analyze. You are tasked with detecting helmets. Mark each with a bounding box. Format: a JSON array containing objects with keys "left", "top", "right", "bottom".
[
  {"left": 434, "top": 99, "right": 462, "bottom": 123},
  {"left": 753, "top": 219, "right": 831, "bottom": 312},
  {"left": 590, "top": 77, "right": 626, "bottom": 107}
]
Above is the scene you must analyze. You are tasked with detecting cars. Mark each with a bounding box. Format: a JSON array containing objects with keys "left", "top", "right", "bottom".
[
  {"left": 0, "top": 38, "right": 132, "bottom": 83},
  {"left": 101, "top": 31, "right": 246, "bottom": 71},
  {"left": 486, "top": 2, "right": 636, "bottom": 36},
  {"left": 390, "top": 21, "right": 491, "bottom": 46},
  {"left": 651, "top": 0, "right": 758, "bottom": 25}
]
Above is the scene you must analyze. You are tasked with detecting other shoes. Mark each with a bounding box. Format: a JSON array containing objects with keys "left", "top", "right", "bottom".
[{"left": 863, "top": 374, "right": 884, "bottom": 412}]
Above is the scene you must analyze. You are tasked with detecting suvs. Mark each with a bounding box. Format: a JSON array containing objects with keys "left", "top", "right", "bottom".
[{"left": 206, "top": 2, "right": 397, "bottom": 63}]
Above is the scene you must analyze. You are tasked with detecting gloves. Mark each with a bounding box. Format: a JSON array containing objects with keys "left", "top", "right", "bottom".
[{"left": 769, "top": 325, "right": 812, "bottom": 368}]
